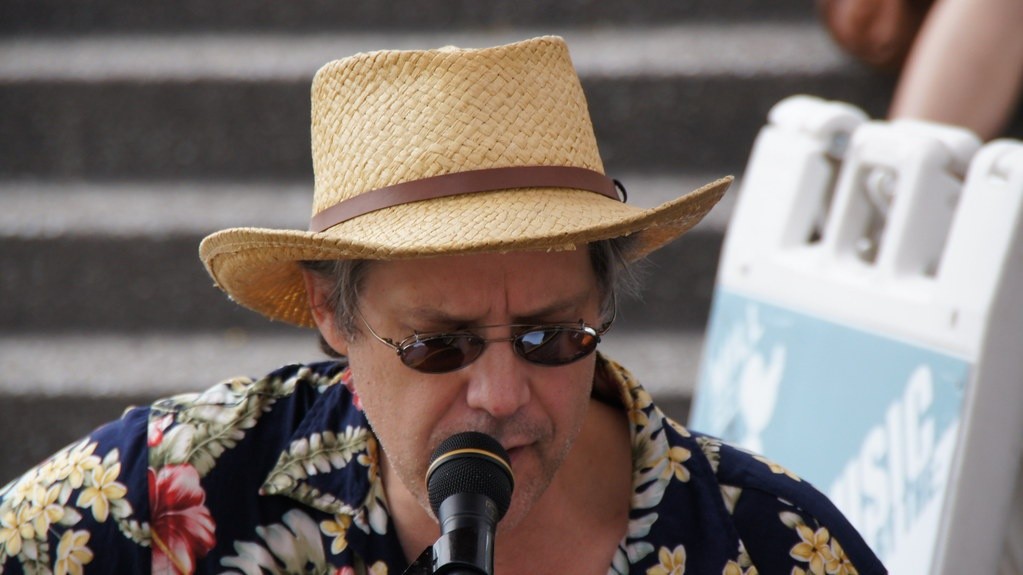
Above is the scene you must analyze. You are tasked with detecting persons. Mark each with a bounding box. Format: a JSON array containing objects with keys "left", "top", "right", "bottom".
[
  {"left": 824, "top": 0, "right": 1023, "bottom": 141},
  {"left": 0, "top": 35, "right": 888, "bottom": 575}
]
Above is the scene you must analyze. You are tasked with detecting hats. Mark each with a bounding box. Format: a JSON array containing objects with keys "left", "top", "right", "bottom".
[{"left": 199, "top": 37, "right": 735, "bottom": 329}]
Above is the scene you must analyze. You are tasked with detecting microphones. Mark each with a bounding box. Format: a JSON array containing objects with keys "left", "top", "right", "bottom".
[{"left": 423, "top": 430, "right": 516, "bottom": 575}]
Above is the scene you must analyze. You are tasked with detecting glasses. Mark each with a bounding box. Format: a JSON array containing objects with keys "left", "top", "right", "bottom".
[{"left": 353, "top": 289, "right": 620, "bottom": 375}]
